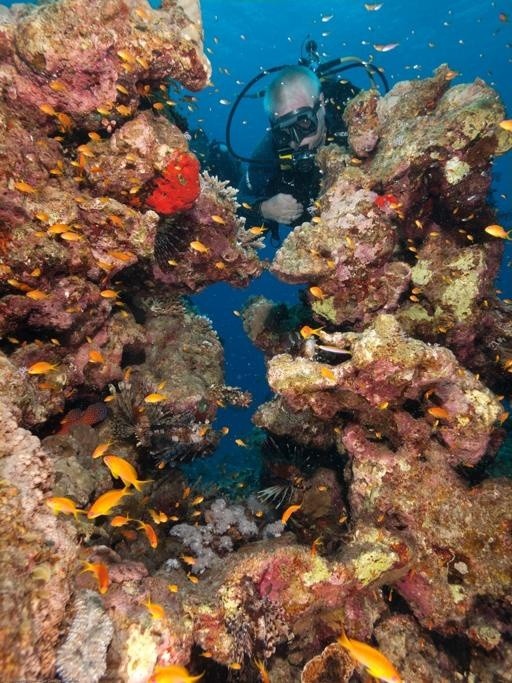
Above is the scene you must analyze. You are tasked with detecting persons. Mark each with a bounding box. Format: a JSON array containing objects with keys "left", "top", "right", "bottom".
[{"left": 236, "top": 65, "right": 348, "bottom": 239}]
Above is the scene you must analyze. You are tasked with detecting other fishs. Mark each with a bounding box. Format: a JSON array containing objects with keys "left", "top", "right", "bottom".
[{"left": 0, "top": 0, "right": 512, "bottom": 683}]
